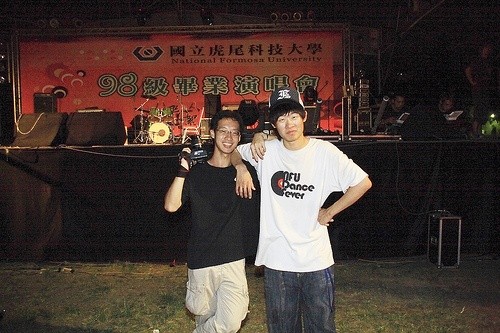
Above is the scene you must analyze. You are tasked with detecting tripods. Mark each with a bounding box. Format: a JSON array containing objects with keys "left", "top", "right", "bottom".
[{"left": 133, "top": 99, "right": 155, "bottom": 144}]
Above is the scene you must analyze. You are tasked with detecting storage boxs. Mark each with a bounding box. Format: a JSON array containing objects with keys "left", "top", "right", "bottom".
[{"left": 427, "top": 214, "right": 462, "bottom": 269}]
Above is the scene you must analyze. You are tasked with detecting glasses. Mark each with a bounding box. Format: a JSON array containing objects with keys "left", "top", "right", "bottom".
[{"left": 215, "top": 129, "right": 240, "bottom": 136}]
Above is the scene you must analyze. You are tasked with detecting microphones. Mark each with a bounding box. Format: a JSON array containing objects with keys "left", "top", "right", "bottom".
[{"left": 142, "top": 94, "right": 157, "bottom": 100}]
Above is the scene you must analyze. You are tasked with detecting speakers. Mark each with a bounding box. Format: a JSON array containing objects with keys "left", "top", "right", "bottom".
[
  {"left": 205, "top": 94, "right": 220, "bottom": 119},
  {"left": 12, "top": 94, "right": 127, "bottom": 148}
]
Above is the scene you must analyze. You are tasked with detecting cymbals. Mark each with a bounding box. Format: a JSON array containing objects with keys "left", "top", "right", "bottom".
[
  {"left": 169, "top": 104, "right": 179, "bottom": 108},
  {"left": 140, "top": 94, "right": 157, "bottom": 100},
  {"left": 182, "top": 108, "right": 193, "bottom": 112}
]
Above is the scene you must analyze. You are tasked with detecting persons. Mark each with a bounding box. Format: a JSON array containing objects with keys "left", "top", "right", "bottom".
[
  {"left": 231, "top": 87, "right": 372, "bottom": 333},
  {"left": 379, "top": 43, "right": 494, "bottom": 138},
  {"left": 164, "top": 110, "right": 279, "bottom": 333}
]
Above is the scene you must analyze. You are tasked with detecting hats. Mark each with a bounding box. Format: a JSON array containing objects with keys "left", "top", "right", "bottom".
[{"left": 268, "top": 86, "right": 305, "bottom": 111}]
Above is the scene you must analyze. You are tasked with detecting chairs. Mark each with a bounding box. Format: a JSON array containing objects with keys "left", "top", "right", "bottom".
[{"left": 182, "top": 107, "right": 204, "bottom": 144}]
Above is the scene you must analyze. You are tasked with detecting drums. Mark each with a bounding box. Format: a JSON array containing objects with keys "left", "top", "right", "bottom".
[
  {"left": 149, "top": 108, "right": 160, "bottom": 114},
  {"left": 163, "top": 108, "right": 172, "bottom": 114},
  {"left": 149, "top": 122, "right": 170, "bottom": 143}
]
